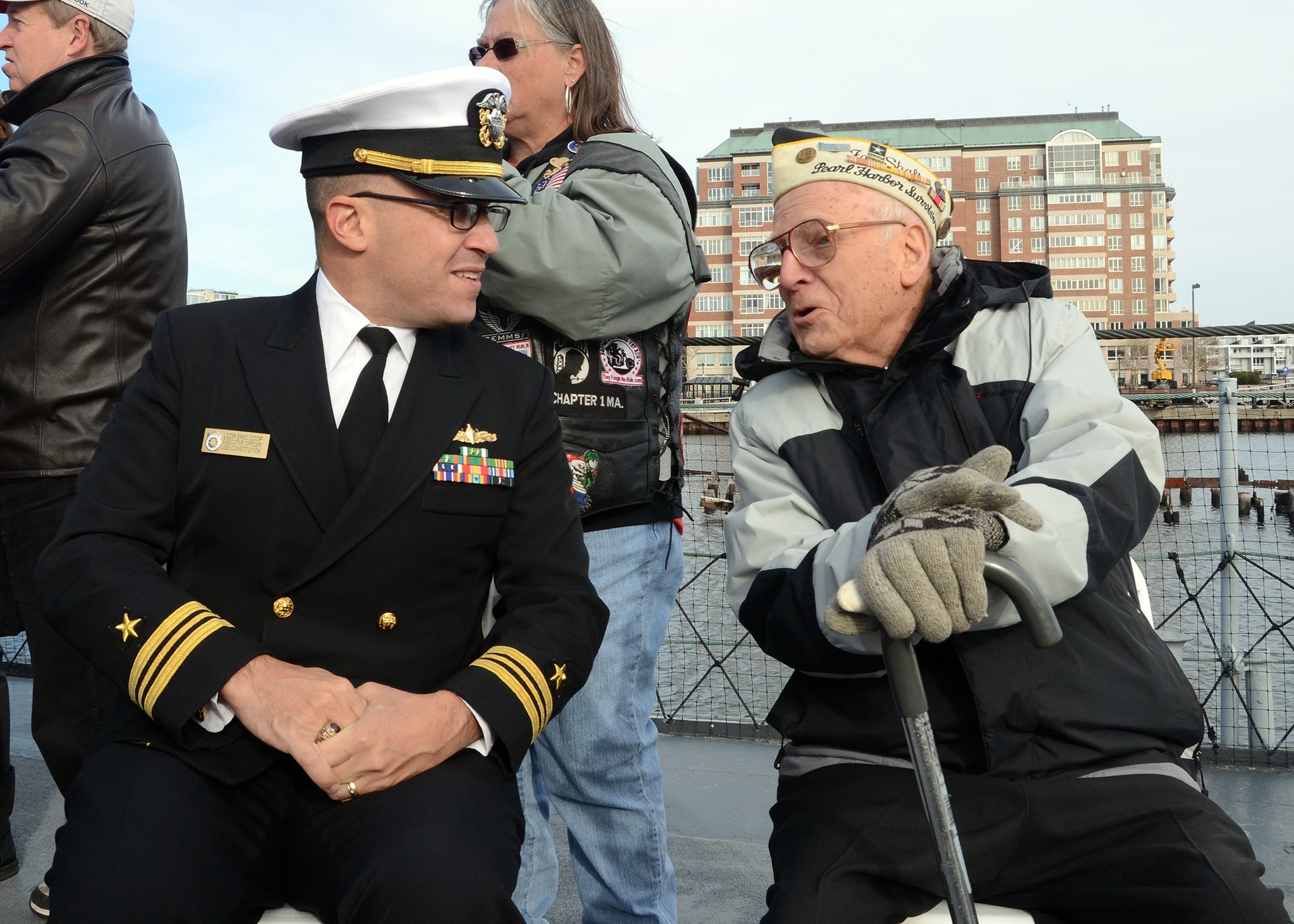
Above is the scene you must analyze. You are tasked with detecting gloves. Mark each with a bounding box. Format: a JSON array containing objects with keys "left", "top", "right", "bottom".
[
  {"left": 868, "top": 445, "right": 1044, "bottom": 545},
  {"left": 826, "top": 505, "right": 1005, "bottom": 643}
]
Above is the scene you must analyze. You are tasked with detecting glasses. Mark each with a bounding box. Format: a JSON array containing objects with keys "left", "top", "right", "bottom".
[
  {"left": 749, "top": 218, "right": 907, "bottom": 292},
  {"left": 468, "top": 37, "right": 553, "bottom": 66},
  {"left": 349, "top": 191, "right": 511, "bottom": 232}
]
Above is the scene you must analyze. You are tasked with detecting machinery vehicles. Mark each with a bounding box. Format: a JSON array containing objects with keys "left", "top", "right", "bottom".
[{"left": 1148, "top": 337, "right": 1177, "bottom": 389}]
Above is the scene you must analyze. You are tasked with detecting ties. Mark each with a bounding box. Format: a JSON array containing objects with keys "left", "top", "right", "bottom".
[{"left": 338, "top": 327, "right": 397, "bottom": 496}]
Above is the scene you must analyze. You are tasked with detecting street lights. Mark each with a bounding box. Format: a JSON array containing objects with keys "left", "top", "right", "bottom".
[
  {"left": 1284, "top": 345, "right": 1292, "bottom": 391},
  {"left": 1192, "top": 283, "right": 1200, "bottom": 406},
  {"left": 1270, "top": 348, "right": 1277, "bottom": 390}
]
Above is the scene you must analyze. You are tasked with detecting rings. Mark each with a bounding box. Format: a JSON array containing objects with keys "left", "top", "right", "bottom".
[
  {"left": 314, "top": 721, "right": 341, "bottom": 745},
  {"left": 347, "top": 781, "right": 360, "bottom": 798}
]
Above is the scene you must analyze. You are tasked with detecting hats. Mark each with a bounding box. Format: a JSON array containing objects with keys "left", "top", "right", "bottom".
[
  {"left": 269, "top": 66, "right": 528, "bottom": 205},
  {"left": 61, "top": 0, "right": 135, "bottom": 39},
  {"left": 771, "top": 127, "right": 955, "bottom": 246}
]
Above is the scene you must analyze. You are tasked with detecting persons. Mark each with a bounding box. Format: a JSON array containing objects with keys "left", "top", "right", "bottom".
[
  {"left": 457, "top": 0, "right": 714, "bottom": 924},
  {"left": 35, "top": 65, "right": 610, "bottom": 924},
  {"left": 0, "top": 0, "right": 187, "bottom": 916},
  {"left": 724, "top": 127, "right": 1294, "bottom": 924}
]
